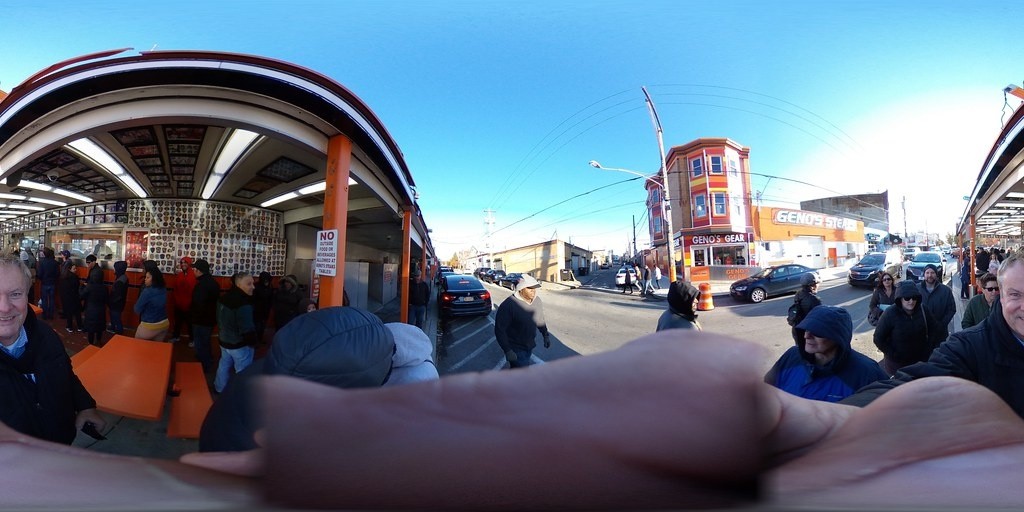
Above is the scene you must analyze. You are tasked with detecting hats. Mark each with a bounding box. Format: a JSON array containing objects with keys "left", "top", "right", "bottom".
[
  {"left": 20, "top": 251, "right": 29, "bottom": 260},
  {"left": 977, "top": 246, "right": 983, "bottom": 249},
  {"left": 527, "top": 284, "right": 541, "bottom": 288},
  {"left": 924, "top": 264, "right": 937, "bottom": 274},
  {"left": 60, "top": 250, "right": 70, "bottom": 257},
  {"left": 190, "top": 260, "right": 209, "bottom": 274},
  {"left": 414, "top": 270, "right": 422, "bottom": 275}
]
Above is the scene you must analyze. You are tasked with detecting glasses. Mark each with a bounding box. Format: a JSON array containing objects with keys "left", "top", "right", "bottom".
[
  {"left": 904, "top": 296, "right": 918, "bottom": 301},
  {"left": 882, "top": 278, "right": 892, "bottom": 281},
  {"left": 984, "top": 287, "right": 999, "bottom": 291},
  {"left": 86, "top": 261, "right": 91, "bottom": 263},
  {"left": 414, "top": 275, "right": 420, "bottom": 277}
]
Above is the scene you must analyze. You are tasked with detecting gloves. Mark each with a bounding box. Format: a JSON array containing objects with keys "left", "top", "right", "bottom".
[
  {"left": 505, "top": 349, "right": 517, "bottom": 361},
  {"left": 544, "top": 335, "right": 550, "bottom": 348}
]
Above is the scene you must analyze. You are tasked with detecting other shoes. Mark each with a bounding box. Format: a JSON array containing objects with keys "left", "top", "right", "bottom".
[
  {"left": 77, "top": 327, "right": 82, "bottom": 332},
  {"left": 58, "top": 311, "right": 65, "bottom": 319},
  {"left": 188, "top": 341, "right": 194, "bottom": 347},
  {"left": 621, "top": 291, "right": 625, "bottom": 293},
  {"left": 168, "top": 338, "right": 180, "bottom": 342},
  {"left": 960, "top": 297, "right": 970, "bottom": 301},
  {"left": 42, "top": 316, "right": 53, "bottom": 320},
  {"left": 630, "top": 292, "right": 633, "bottom": 294},
  {"left": 251, "top": 340, "right": 266, "bottom": 344},
  {"left": 106, "top": 328, "right": 123, "bottom": 333},
  {"left": 65, "top": 328, "right": 74, "bottom": 332}
]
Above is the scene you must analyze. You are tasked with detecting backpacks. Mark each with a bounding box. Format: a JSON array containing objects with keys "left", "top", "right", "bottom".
[{"left": 787, "top": 291, "right": 812, "bottom": 326}]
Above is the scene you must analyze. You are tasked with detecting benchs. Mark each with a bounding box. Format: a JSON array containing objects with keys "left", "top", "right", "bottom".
[
  {"left": 166, "top": 362, "right": 219, "bottom": 436},
  {"left": 67, "top": 342, "right": 102, "bottom": 369}
]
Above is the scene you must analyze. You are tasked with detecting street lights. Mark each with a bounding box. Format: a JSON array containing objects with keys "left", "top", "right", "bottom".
[{"left": 589, "top": 159, "right": 677, "bottom": 287}]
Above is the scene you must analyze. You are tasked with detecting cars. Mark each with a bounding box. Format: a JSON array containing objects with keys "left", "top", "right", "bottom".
[
  {"left": 730, "top": 263, "right": 822, "bottom": 303},
  {"left": 846, "top": 243, "right": 960, "bottom": 289},
  {"left": 601, "top": 262, "right": 609, "bottom": 269},
  {"left": 475, "top": 267, "right": 524, "bottom": 290},
  {"left": 436, "top": 265, "right": 492, "bottom": 318},
  {"left": 615, "top": 262, "right": 639, "bottom": 285}
]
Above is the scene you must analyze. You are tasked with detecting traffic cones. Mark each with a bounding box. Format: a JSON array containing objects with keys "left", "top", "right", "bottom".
[{"left": 698, "top": 283, "right": 714, "bottom": 312}]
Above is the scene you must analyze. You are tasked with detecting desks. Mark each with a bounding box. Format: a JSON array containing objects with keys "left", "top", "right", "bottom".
[{"left": 71, "top": 335, "right": 176, "bottom": 421}]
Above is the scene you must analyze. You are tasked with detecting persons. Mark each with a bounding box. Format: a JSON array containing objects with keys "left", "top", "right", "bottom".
[
  {"left": 0, "top": 253, "right": 107, "bottom": 446},
  {"left": 411, "top": 259, "right": 430, "bottom": 277},
  {"left": 197, "top": 307, "right": 396, "bottom": 453},
  {"left": 762, "top": 244, "right": 1024, "bottom": 458},
  {"left": 214, "top": 273, "right": 259, "bottom": 393},
  {"left": 655, "top": 280, "right": 703, "bottom": 332},
  {"left": 961, "top": 271, "right": 998, "bottom": 329},
  {"left": 16, "top": 238, "right": 351, "bottom": 346},
  {"left": 960, "top": 246, "right": 1015, "bottom": 299},
  {"left": 383, "top": 321, "right": 441, "bottom": 388},
  {"left": 763, "top": 305, "right": 889, "bottom": 403},
  {"left": 869, "top": 272, "right": 896, "bottom": 318},
  {"left": 621, "top": 267, "right": 633, "bottom": 295},
  {"left": 494, "top": 276, "right": 551, "bottom": 369},
  {"left": 191, "top": 259, "right": 222, "bottom": 371},
  {"left": 409, "top": 270, "right": 430, "bottom": 329},
  {"left": 873, "top": 280, "right": 940, "bottom": 376},
  {"left": 916, "top": 264, "right": 956, "bottom": 360},
  {"left": 634, "top": 264, "right": 662, "bottom": 295},
  {"left": 791, "top": 271, "right": 822, "bottom": 346}
]
